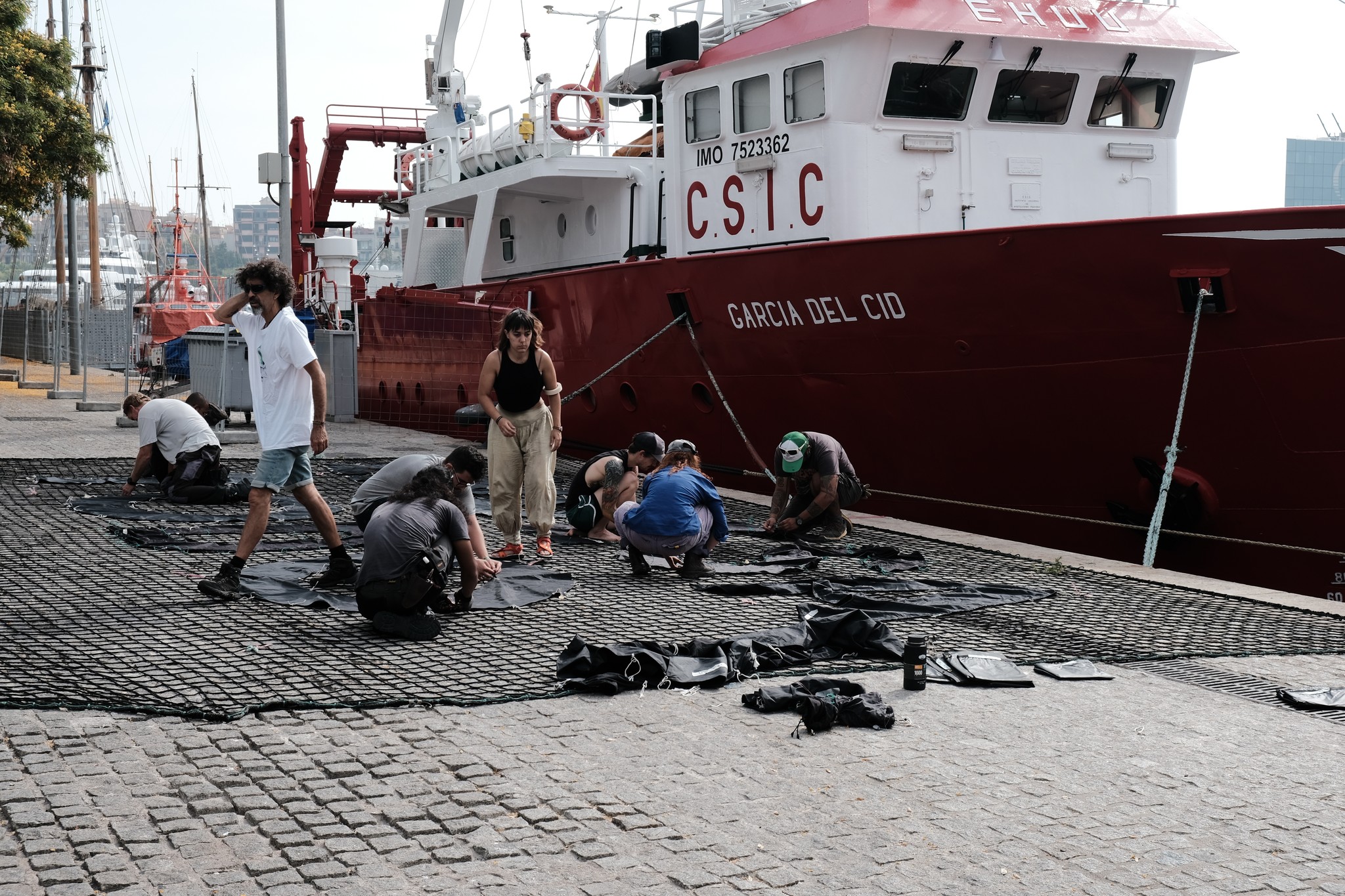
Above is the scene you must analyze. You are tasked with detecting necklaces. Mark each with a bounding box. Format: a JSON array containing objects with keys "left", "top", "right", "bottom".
[{"left": 510, "top": 348, "right": 527, "bottom": 363}]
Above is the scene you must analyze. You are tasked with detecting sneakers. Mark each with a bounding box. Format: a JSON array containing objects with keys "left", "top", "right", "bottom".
[
  {"left": 198, "top": 562, "right": 242, "bottom": 599},
  {"left": 309, "top": 555, "right": 358, "bottom": 587}
]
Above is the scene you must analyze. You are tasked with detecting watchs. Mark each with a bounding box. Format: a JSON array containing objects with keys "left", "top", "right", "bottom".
[
  {"left": 553, "top": 426, "right": 563, "bottom": 432},
  {"left": 795, "top": 516, "right": 803, "bottom": 525},
  {"left": 127, "top": 477, "right": 137, "bottom": 486}
]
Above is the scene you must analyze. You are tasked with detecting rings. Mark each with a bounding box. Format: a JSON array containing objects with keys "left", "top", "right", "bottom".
[{"left": 482, "top": 576, "right": 485, "bottom": 578}]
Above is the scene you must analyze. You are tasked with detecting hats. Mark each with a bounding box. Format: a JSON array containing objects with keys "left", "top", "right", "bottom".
[
  {"left": 779, "top": 431, "right": 808, "bottom": 472},
  {"left": 666, "top": 439, "right": 698, "bottom": 454},
  {"left": 632, "top": 432, "right": 666, "bottom": 462}
]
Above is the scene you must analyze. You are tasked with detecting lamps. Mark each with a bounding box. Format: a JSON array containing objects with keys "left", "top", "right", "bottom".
[{"left": 297, "top": 232, "right": 317, "bottom": 243}]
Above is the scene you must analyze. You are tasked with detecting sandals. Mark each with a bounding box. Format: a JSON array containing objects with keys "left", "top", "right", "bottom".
[
  {"left": 489, "top": 543, "right": 524, "bottom": 560},
  {"left": 536, "top": 537, "right": 553, "bottom": 559}
]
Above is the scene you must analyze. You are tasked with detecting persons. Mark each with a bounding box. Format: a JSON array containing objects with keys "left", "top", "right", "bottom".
[
  {"left": 476, "top": 307, "right": 562, "bottom": 560},
  {"left": 121, "top": 392, "right": 252, "bottom": 503},
  {"left": 565, "top": 432, "right": 666, "bottom": 541},
  {"left": 613, "top": 439, "right": 730, "bottom": 578},
  {"left": 350, "top": 445, "right": 502, "bottom": 582},
  {"left": 197, "top": 259, "right": 360, "bottom": 599},
  {"left": 354, "top": 466, "right": 478, "bottom": 640},
  {"left": 763, "top": 431, "right": 864, "bottom": 540}
]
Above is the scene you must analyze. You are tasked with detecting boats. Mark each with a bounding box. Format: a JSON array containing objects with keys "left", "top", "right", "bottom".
[
  {"left": 0, "top": 215, "right": 208, "bottom": 324},
  {"left": 131, "top": 154, "right": 239, "bottom": 379},
  {"left": 293, "top": 0, "right": 1345, "bottom": 601}
]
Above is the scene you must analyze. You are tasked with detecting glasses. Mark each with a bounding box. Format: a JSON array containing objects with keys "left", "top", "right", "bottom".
[
  {"left": 193, "top": 404, "right": 208, "bottom": 417},
  {"left": 451, "top": 465, "right": 475, "bottom": 488},
  {"left": 779, "top": 440, "right": 807, "bottom": 456},
  {"left": 243, "top": 284, "right": 270, "bottom": 293}
]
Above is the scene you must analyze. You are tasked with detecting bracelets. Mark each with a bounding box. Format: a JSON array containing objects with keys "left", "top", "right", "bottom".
[
  {"left": 483, "top": 557, "right": 491, "bottom": 560},
  {"left": 496, "top": 416, "right": 503, "bottom": 425},
  {"left": 313, "top": 420, "right": 332, "bottom": 432},
  {"left": 769, "top": 516, "right": 778, "bottom": 522}
]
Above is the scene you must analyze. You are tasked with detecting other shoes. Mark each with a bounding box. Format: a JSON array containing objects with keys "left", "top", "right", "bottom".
[
  {"left": 217, "top": 463, "right": 230, "bottom": 484},
  {"left": 236, "top": 477, "right": 252, "bottom": 497},
  {"left": 683, "top": 553, "right": 716, "bottom": 577},
  {"left": 373, "top": 610, "right": 443, "bottom": 640},
  {"left": 629, "top": 554, "right": 652, "bottom": 576},
  {"left": 842, "top": 512, "right": 853, "bottom": 533},
  {"left": 817, "top": 517, "right": 847, "bottom": 540}
]
